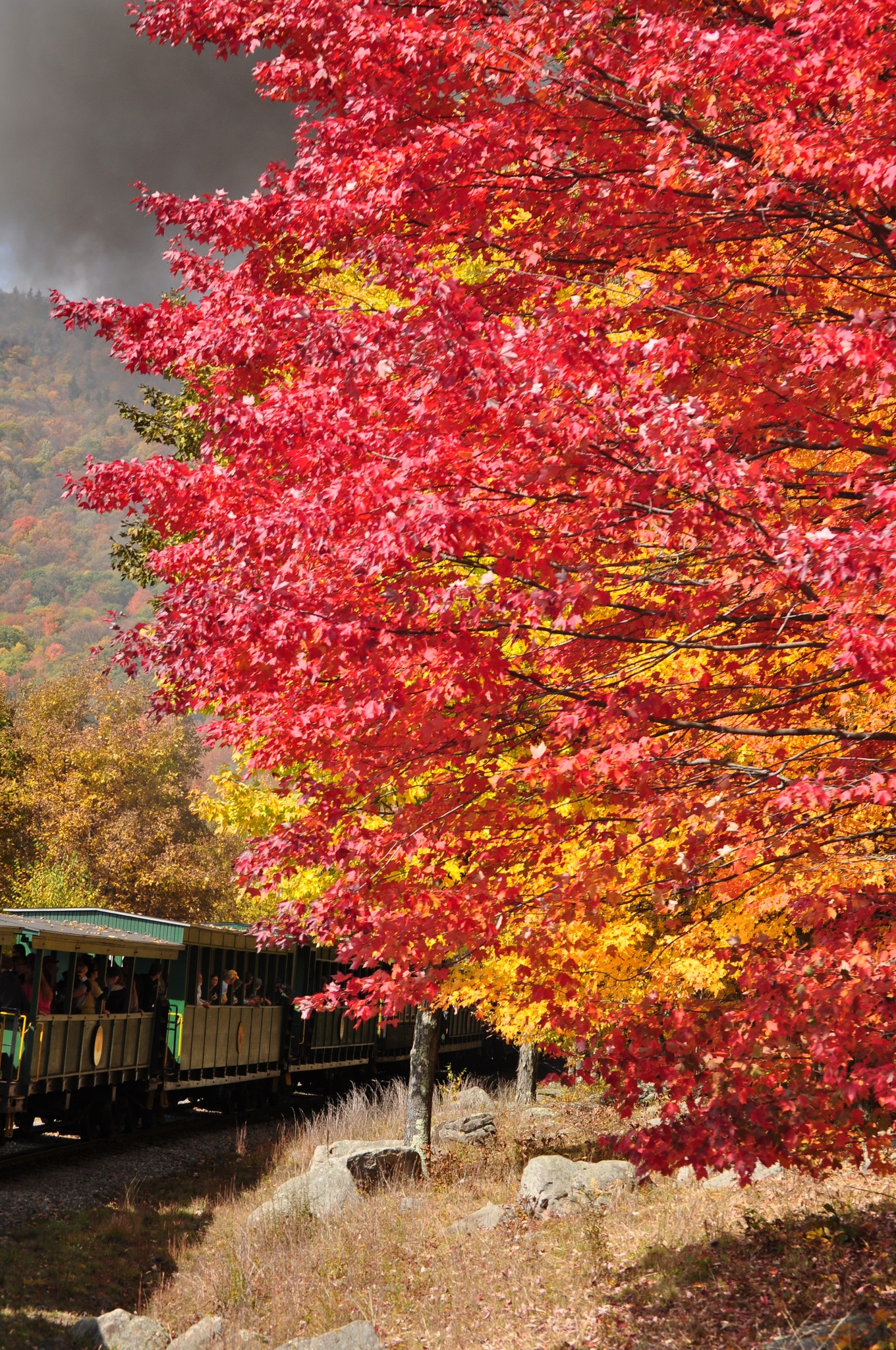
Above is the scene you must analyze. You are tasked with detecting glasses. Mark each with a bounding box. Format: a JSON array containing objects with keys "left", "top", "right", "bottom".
[{"left": 279, "top": 981, "right": 282, "bottom": 983}]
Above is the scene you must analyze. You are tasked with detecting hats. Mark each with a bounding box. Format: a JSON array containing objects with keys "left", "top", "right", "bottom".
[
  {"left": 255, "top": 978, "right": 263, "bottom": 986},
  {"left": 229, "top": 970, "right": 239, "bottom": 979},
  {"left": 44, "top": 956, "right": 59, "bottom": 964}
]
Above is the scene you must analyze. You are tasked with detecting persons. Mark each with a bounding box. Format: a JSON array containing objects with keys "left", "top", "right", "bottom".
[{"left": 0, "top": 942, "right": 326, "bottom": 1017}]
